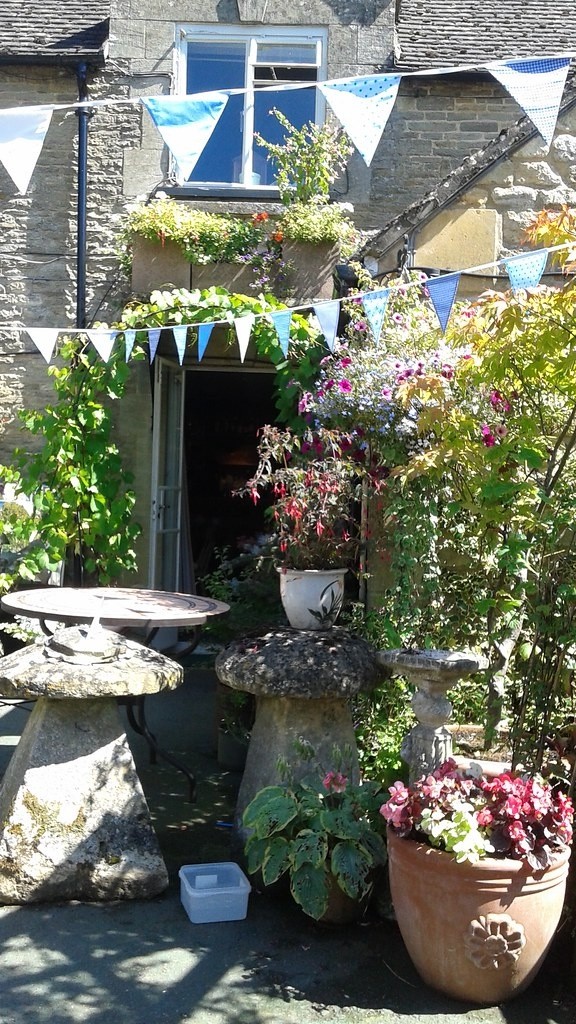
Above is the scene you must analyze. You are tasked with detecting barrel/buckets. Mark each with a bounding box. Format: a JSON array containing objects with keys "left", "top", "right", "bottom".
[{"left": 141, "top": 643, "right": 225, "bottom": 754}]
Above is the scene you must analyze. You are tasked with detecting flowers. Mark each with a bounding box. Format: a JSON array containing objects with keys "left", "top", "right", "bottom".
[
  {"left": 240, "top": 732, "right": 390, "bottom": 923},
  {"left": 231, "top": 422, "right": 398, "bottom": 579},
  {"left": 380, "top": 757, "right": 575, "bottom": 870}
]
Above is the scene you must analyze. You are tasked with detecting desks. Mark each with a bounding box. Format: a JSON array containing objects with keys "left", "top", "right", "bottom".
[{"left": 0, "top": 586, "right": 232, "bottom": 805}]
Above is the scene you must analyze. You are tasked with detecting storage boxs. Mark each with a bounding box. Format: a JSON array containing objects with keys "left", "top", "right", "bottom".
[{"left": 178, "top": 862, "right": 252, "bottom": 924}]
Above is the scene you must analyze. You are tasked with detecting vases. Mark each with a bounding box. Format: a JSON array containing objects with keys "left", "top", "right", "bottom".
[
  {"left": 387, "top": 772, "right": 571, "bottom": 1006},
  {"left": 317, "top": 858, "right": 354, "bottom": 922},
  {"left": 275, "top": 566, "right": 348, "bottom": 630}
]
